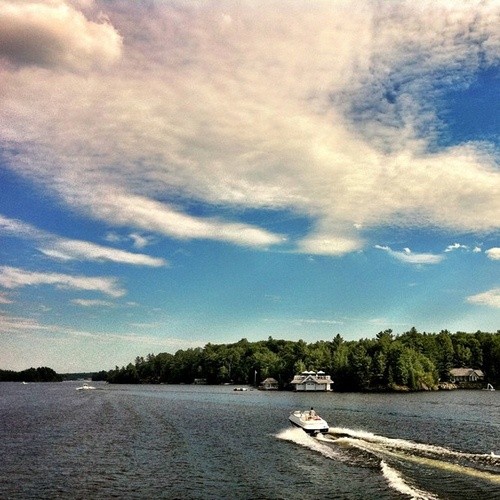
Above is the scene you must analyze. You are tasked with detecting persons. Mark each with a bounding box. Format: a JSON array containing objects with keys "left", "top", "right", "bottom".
[{"left": 309, "top": 407, "right": 316, "bottom": 416}]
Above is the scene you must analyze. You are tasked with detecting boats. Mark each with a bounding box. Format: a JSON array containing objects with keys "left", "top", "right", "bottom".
[
  {"left": 20, "top": 381, "right": 29, "bottom": 385},
  {"left": 288, "top": 408, "right": 331, "bottom": 435},
  {"left": 481, "top": 388, "right": 496, "bottom": 392},
  {"left": 234, "top": 387, "right": 248, "bottom": 391},
  {"left": 76, "top": 385, "right": 96, "bottom": 392}
]
